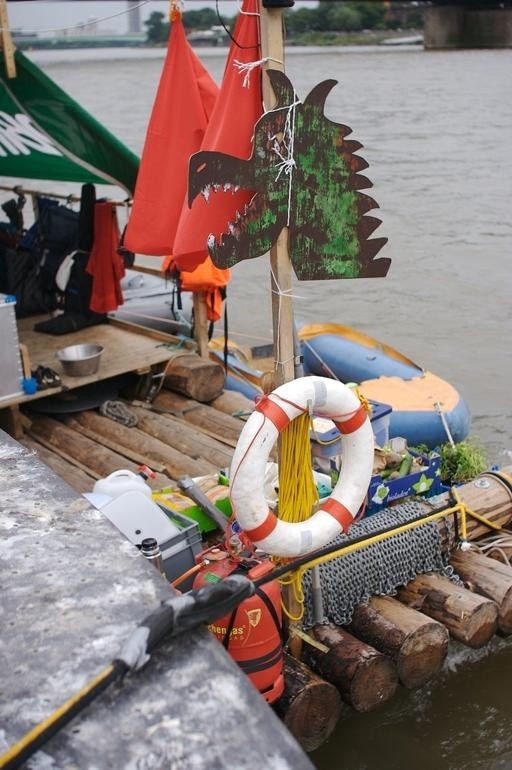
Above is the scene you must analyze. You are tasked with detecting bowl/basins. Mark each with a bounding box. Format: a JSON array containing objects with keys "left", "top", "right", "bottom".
[{"left": 54, "top": 343, "right": 105, "bottom": 377}]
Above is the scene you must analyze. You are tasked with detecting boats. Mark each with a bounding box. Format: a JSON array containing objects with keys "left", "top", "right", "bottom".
[
  {"left": 1, "top": 35, "right": 512, "bottom": 753},
  {"left": 209, "top": 329, "right": 468, "bottom": 448}
]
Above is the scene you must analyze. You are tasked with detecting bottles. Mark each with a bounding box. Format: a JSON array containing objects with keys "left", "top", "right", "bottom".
[{"left": 139, "top": 538, "right": 167, "bottom": 578}]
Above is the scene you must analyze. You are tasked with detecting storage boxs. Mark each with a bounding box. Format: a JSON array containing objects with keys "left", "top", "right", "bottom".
[
  {"left": 133, "top": 502, "right": 204, "bottom": 596},
  {"left": 308, "top": 401, "right": 392, "bottom": 479}
]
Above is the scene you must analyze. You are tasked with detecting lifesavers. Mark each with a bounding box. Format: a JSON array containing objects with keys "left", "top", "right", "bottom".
[{"left": 228, "top": 376, "right": 375, "bottom": 558}]
